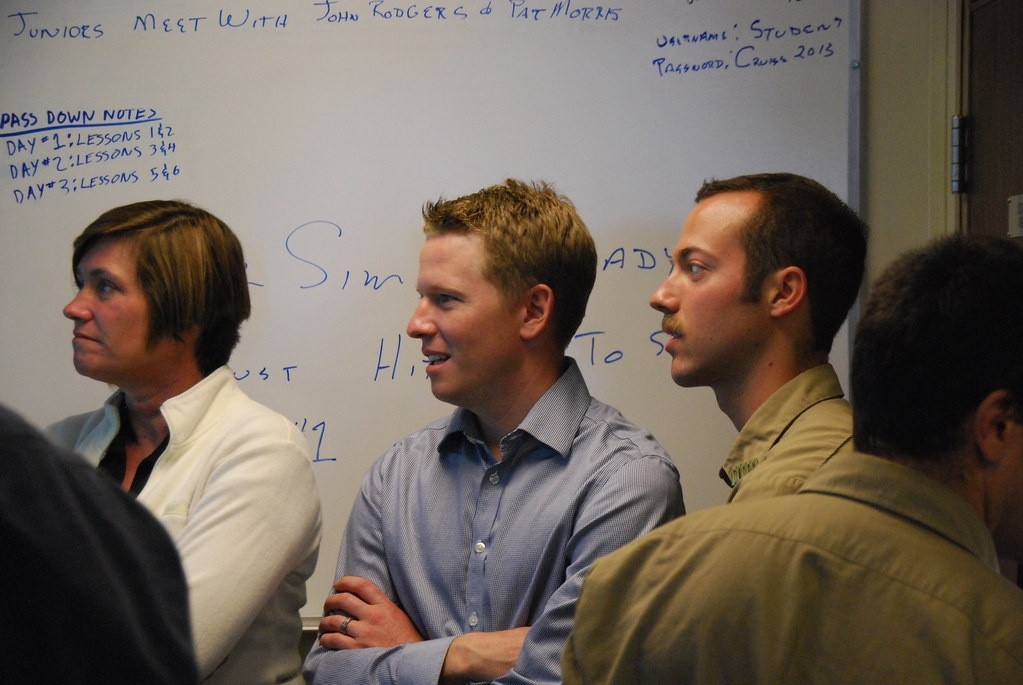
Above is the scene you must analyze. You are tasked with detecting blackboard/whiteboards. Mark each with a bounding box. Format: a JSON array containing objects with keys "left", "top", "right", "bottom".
[{"left": 0, "top": 0, "right": 865, "bottom": 631}]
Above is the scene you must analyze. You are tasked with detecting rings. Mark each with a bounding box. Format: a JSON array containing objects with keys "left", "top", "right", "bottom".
[{"left": 340, "top": 616, "right": 355, "bottom": 635}]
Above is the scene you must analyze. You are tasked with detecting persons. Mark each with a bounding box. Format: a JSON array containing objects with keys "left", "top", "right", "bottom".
[
  {"left": 558, "top": 233, "right": 1022, "bottom": 685},
  {"left": 648, "top": 171, "right": 870, "bottom": 505},
  {"left": 40, "top": 197, "right": 322, "bottom": 685},
  {"left": 0, "top": 402, "right": 201, "bottom": 685},
  {"left": 300, "top": 178, "right": 686, "bottom": 685}
]
